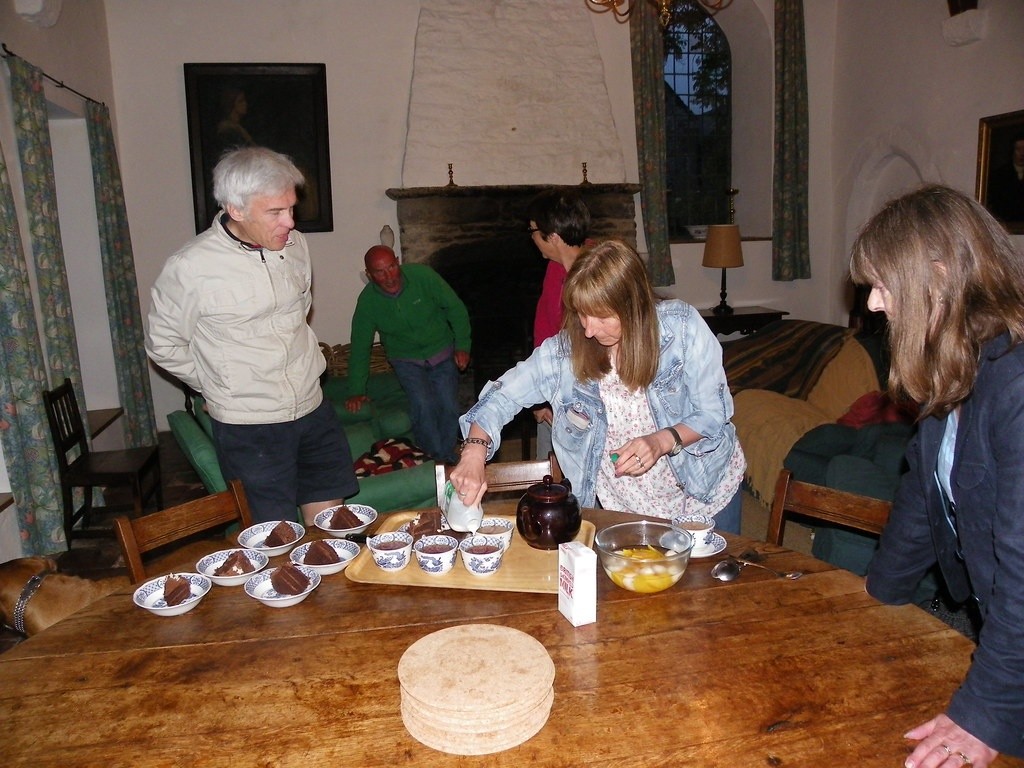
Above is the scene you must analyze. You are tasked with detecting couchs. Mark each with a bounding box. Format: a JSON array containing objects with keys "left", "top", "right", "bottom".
[
  {"left": 165, "top": 371, "right": 438, "bottom": 537},
  {"left": 721, "top": 317, "right": 981, "bottom": 638}
]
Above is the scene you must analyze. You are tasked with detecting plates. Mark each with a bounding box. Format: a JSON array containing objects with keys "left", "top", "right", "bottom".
[{"left": 659, "top": 530, "right": 727, "bottom": 559}]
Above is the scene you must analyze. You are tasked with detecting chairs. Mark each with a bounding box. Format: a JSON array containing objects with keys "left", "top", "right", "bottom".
[
  {"left": 41, "top": 377, "right": 165, "bottom": 551},
  {"left": 435, "top": 450, "right": 565, "bottom": 508},
  {"left": 112, "top": 478, "right": 254, "bottom": 585},
  {"left": 765, "top": 469, "right": 894, "bottom": 547}
]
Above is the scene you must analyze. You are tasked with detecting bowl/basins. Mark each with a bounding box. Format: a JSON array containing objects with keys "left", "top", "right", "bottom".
[
  {"left": 595, "top": 521, "right": 695, "bottom": 594},
  {"left": 132, "top": 503, "right": 378, "bottom": 617}
]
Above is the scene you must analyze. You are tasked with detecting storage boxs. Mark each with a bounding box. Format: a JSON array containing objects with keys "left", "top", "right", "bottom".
[{"left": 557, "top": 540, "right": 597, "bottom": 628}]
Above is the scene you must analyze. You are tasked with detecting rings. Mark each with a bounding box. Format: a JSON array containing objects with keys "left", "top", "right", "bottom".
[
  {"left": 940, "top": 744, "right": 951, "bottom": 755},
  {"left": 643, "top": 466, "right": 648, "bottom": 472},
  {"left": 639, "top": 461, "right": 644, "bottom": 468},
  {"left": 957, "top": 751, "right": 969, "bottom": 764},
  {"left": 458, "top": 490, "right": 467, "bottom": 496},
  {"left": 634, "top": 455, "right": 641, "bottom": 463}
]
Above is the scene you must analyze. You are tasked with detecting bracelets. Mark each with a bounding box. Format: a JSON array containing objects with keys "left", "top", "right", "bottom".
[{"left": 459, "top": 438, "right": 489, "bottom": 455}]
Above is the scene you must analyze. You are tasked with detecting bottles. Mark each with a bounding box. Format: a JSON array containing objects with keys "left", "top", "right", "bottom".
[{"left": 441, "top": 480, "right": 484, "bottom": 533}]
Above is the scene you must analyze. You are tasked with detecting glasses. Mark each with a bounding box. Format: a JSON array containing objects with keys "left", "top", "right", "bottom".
[{"left": 527, "top": 226, "right": 543, "bottom": 237}]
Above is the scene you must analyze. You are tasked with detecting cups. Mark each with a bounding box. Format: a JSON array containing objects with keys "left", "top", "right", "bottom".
[
  {"left": 367, "top": 516, "right": 515, "bottom": 577},
  {"left": 672, "top": 513, "right": 715, "bottom": 550}
]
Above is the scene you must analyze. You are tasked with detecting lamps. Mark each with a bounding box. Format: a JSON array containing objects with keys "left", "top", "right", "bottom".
[
  {"left": 584, "top": 0, "right": 732, "bottom": 28},
  {"left": 702, "top": 225, "right": 744, "bottom": 315}
]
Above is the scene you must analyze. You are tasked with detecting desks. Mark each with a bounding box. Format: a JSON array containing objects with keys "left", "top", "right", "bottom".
[{"left": 0, "top": 498, "right": 1024, "bottom": 768}]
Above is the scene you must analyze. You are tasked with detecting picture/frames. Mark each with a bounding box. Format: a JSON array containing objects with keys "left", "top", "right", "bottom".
[
  {"left": 975, "top": 110, "right": 1024, "bottom": 237},
  {"left": 182, "top": 62, "right": 334, "bottom": 236}
]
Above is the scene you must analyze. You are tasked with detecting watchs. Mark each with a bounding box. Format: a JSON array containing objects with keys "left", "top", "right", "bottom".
[{"left": 663, "top": 427, "right": 683, "bottom": 457}]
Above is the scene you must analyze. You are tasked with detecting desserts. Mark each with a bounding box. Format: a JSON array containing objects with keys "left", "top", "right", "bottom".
[{"left": 164, "top": 506, "right": 363, "bottom": 606}]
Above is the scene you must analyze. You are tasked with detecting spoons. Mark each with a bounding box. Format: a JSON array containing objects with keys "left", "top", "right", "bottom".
[
  {"left": 710, "top": 548, "right": 759, "bottom": 582},
  {"left": 730, "top": 553, "right": 802, "bottom": 580}
]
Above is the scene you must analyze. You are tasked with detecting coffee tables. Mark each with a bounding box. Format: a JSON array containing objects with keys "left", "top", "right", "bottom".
[{"left": 698, "top": 306, "right": 791, "bottom": 336}]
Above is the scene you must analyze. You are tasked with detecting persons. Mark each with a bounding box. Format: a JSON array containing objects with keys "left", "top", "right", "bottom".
[
  {"left": 451, "top": 240, "right": 747, "bottom": 534},
  {"left": 850, "top": 182, "right": 1024, "bottom": 768},
  {"left": 526, "top": 187, "right": 595, "bottom": 458},
  {"left": 145, "top": 142, "right": 361, "bottom": 538},
  {"left": 345, "top": 245, "right": 472, "bottom": 466}
]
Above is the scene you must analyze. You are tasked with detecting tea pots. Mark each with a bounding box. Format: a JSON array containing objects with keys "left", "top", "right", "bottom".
[{"left": 515, "top": 474, "right": 582, "bottom": 550}]
